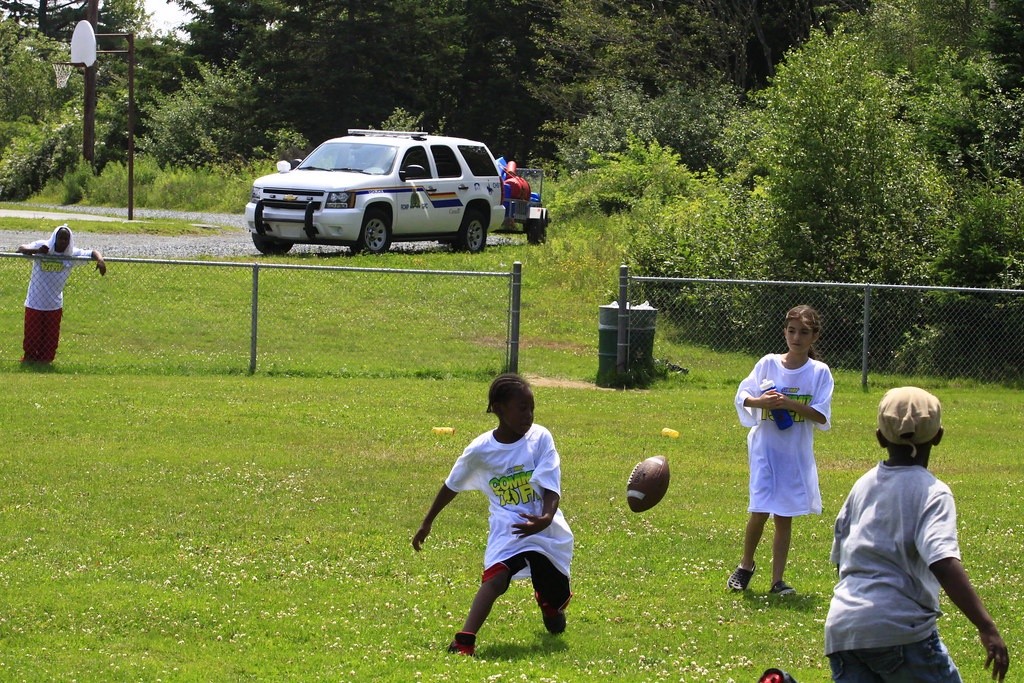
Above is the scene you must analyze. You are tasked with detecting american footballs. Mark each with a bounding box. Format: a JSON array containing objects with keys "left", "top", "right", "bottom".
[{"left": 625, "top": 455, "right": 671, "bottom": 512}]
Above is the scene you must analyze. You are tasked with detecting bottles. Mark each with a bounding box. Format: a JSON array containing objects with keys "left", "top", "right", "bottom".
[{"left": 759, "top": 378, "right": 794, "bottom": 431}]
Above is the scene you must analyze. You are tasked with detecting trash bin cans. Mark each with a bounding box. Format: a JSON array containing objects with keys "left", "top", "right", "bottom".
[{"left": 595, "top": 299, "right": 658, "bottom": 390}]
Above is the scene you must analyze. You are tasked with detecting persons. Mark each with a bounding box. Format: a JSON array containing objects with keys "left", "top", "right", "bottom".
[
  {"left": 824, "top": 385, "right": 1009, "bottom": 683},
  {"left": 17, "top": 225, "right": 105, "bottom": 362},
  {"left": 726, "top": 304, "right": 834, "bottom": 595},
  {"left": 411, "top": 372, "right": 574, "bottom": 657}
]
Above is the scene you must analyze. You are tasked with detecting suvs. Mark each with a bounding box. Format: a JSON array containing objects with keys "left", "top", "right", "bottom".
[{"left": 244, "top": 128, "right": 506, "bottom": 259}]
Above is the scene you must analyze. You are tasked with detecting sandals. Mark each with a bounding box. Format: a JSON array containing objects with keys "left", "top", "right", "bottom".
[
  {"left": 768, "top": 581, "right": 798, "bottom": 598},
  {"left": 726, "top": 557, "right": 756, "bottom": 592}
]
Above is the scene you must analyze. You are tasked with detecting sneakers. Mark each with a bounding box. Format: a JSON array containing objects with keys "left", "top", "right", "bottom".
[
  {"left": 542, "top": 607, "right": 568, "bottom": 634},
  {"left": 447, "top": 628, "right": 477, "bottom": 660}
]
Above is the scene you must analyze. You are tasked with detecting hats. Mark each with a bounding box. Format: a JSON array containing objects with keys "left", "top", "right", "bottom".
[{"left": 876, "top": 382, "right": 942, "bottom": 461}]
